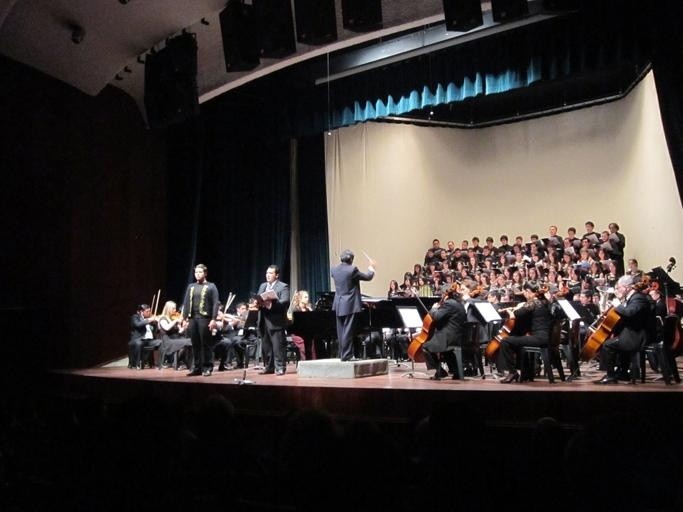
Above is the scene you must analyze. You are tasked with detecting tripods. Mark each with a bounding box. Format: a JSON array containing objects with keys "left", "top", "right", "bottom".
[
  {"left": 554, "top": 321, "right": 582, "bottom": 380},
  {"left": 233, "top": 345, "right": 256, "bottom": 385},
  {"left": 399, "top": 328, "right": 427, "bottom": 378},
  {"left": 388, "top": 328, "right": 410, "bottom": 369},
  {"left": 474, "top": 324, "right": 506, "bottom": 380}
]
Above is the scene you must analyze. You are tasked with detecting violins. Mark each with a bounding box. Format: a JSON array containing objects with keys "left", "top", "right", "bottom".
[{"left": 216, "top": 313, "right": 244, "bottom": 323}]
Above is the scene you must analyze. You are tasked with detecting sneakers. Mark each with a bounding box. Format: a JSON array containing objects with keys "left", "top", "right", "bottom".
[
  {"left": 345, "top": 355, "right": 360, "bottom": 361},
  {"left": 186, "top": 370, "right": 202, "bottom": 375},
  {"left": 203, "top": 371, "right": 212, "bottom": 375},
  {"left": 259, "top": 369, "right": 275, "bottom": 374},
  {"left": 128, "top": 362, "right": 249, "bottom": 370},
  {"left": 276, "top": 369, "right": 286, "bottom": 375}
]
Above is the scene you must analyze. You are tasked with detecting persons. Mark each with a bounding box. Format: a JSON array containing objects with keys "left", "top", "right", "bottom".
[
  {"left": 249, "top": 265, "right": 290, "bottom": 375},
  {"left": 126, "top": 289, "right": 324, "bottom": 371},
  {"left": 385, "top": 223, "right": 665, "bottom": 385},
  {"left": 179, "top": 263, "right": 219, "bottom": 377},
  {"left": 328, "top": 249, "right": 375, "bottom": 362}
]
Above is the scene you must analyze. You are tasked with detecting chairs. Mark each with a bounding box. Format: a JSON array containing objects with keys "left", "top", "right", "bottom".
[
  {"left": 140, "top": 336, "right": 302, "bottom": 372},
  {"left": 433, "top": 311, "right": 682, "bottom": 389}
]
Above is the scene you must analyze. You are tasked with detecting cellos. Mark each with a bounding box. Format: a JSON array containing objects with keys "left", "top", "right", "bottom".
[
  {"left": 660, "top": 257, "right": 683, "bottom": 358},
  {"left": 407, "top": 283, "right": 456, "bottom": 362},
  {"left": 581, "top": 276, "right": 650, "bottom": 360},
  {"left": 485, "top": 286, "right": 549, "bottom": 358}
]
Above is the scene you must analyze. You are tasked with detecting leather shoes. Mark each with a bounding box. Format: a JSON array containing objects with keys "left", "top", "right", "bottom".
[{"left": 429, "top": 366, "right": 683, "bottom": 385}]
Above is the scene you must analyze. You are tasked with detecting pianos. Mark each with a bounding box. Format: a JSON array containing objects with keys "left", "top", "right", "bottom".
[{"left": 293, "top": 292, "right": 374, "bottom": 360}]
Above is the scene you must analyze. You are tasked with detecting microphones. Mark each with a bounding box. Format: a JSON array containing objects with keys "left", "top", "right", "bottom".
[{"left": 244, "top": 326, "right": 256, "bottom": 339}]
